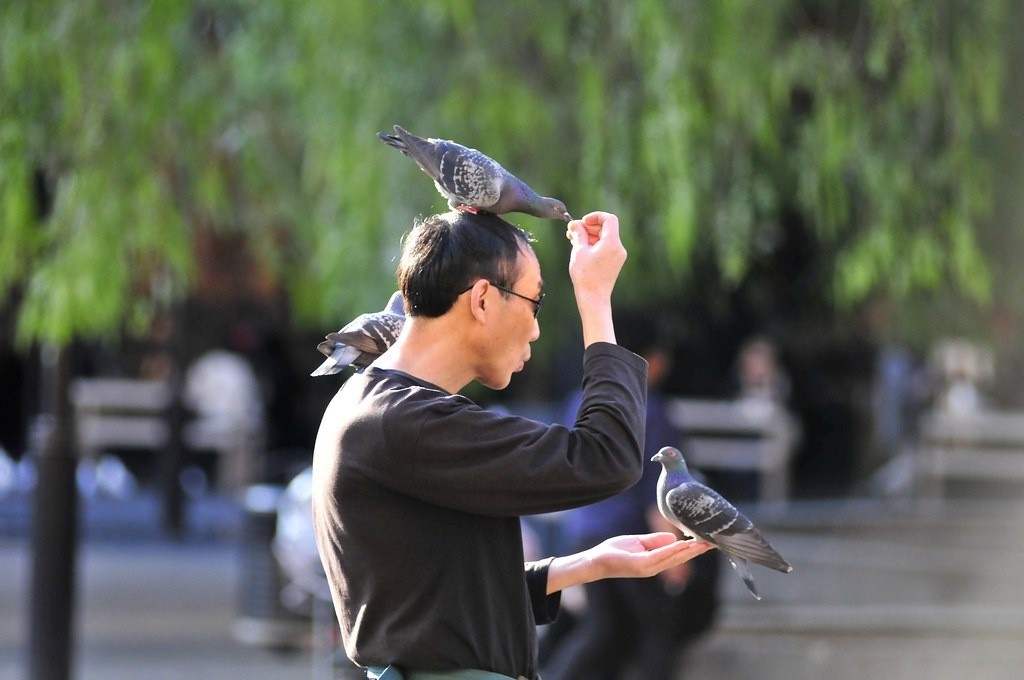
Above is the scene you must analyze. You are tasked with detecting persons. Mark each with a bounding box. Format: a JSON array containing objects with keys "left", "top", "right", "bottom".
[
  {"left": 312, "top": 206, "right": 649, "bottom": 680},
  {"left": 519, "top": 341, "right": 725, "bottom": 680}
]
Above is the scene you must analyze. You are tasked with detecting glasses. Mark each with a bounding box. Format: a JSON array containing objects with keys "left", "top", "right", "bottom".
[{"left": 460, "top": 281, "right": 546, "bottom": 320}]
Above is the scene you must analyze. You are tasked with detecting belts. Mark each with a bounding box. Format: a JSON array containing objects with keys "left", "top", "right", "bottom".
[{"left": 366, "top": 665, "right": 517, "bottom": 680}]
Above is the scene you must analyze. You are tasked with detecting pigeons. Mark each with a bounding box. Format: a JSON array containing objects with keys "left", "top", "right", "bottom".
[
  {"left": 310, "top": 289, "right": 406, "bottom": 377},
  {"left": 376, "top": 124, "right": 576, "bottom": 222},
  {"left": 651, "top": 445, "right": 794, "bottom": 601}
]
